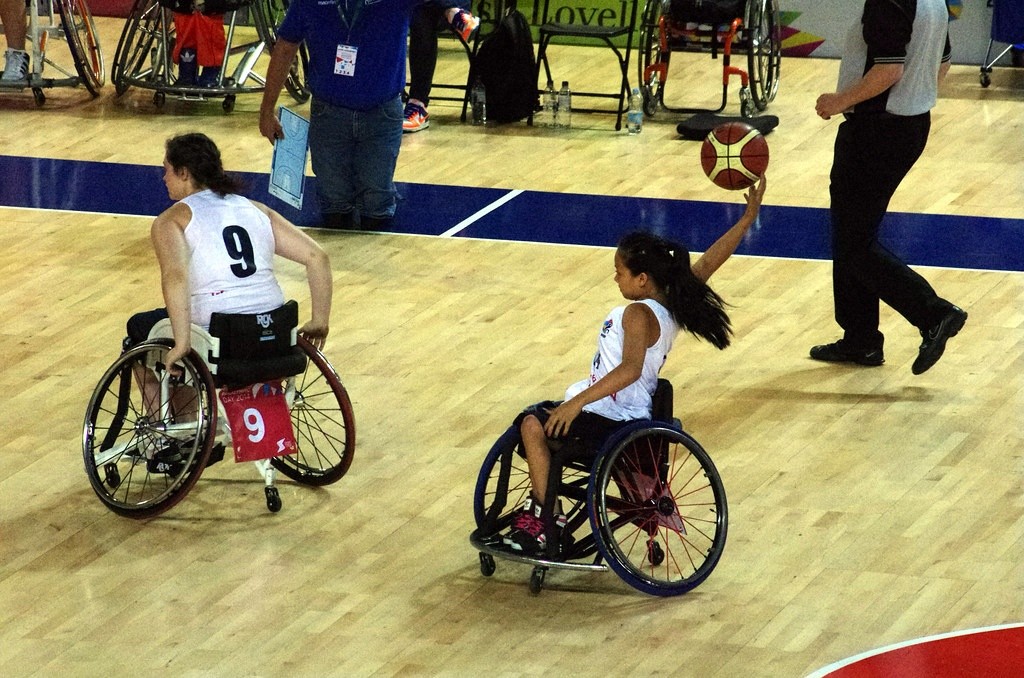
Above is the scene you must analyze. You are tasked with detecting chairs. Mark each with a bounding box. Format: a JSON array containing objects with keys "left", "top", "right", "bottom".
[
  {"left": 399, "top": 0, "right": 503, "bottom": 126},
  {"left": 526, "top": 0, "right": 639, "bottom": 133}
]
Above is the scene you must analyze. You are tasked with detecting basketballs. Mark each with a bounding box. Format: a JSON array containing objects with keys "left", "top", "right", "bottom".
[{"left": 699, "top": 121, "right": 771, "bottom": 190}]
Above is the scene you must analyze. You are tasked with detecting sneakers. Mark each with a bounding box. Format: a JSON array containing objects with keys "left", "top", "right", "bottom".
[
  {"left": 810, "top": 339, "right": 885, "bottom": 366},
  {"left": 126, "top": 440, "right": 182, "bottom": 462},
  {"left": 2, "top": 50, "right": 30, "bottom": 82},
  {"left": 504, "top": 496, "right": 539, "bottom": 544},
  {"left": 511, "top": 506, "right": 568, "bottom": 550},
  {"left": 402, "top": 103, "right": 429, "bottom": 131},
  {"left": 172, "top": 68, "right": 197, "bottom": 87},
  {"left": 177, "top": 435, "right": 203, "bottom": 454},
  {"left": 912, "top": 303, "right": 969, "bottom": 374},
  {"left": 450, "top": 8, "right": 482, "bottom": 43},
  {"left": 198, "top": 66, "right": 221, "bottom": 87}
]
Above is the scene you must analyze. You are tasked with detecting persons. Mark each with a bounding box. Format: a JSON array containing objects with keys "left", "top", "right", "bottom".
[
  {"left": 123, "top": 133, "right": 332, "bottom": 459},
  {"left": 0, "top": 0, "right": 30, "bottom": 81},
  {"left": 503, "top": 169, "right": 766, "bottom": 554},
  {"left": 809, "top": 0, "right": 967, "bottom": 374},
  {"left": 402, "top": 0, "right": 482, "bottom": 130},
  {"left": 259, "top": 0, "right": 414, "bottom": 218}
]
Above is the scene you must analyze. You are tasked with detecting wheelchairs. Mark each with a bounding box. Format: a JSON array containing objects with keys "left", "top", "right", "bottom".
[
  {"left": 469, "top": 378, "right": 728, "bottom": 597},
  {"left": 640, "top": 0, "right": 782, "bottom": 120},
  {"left": 0, "top": 0, "right": 106, "bottom": 106},
  {"left": 110, "top": 0, "right": 311, "bottom": 113},
  {"left": 81, "top": 299, "right": 357, "bottom": 520}
]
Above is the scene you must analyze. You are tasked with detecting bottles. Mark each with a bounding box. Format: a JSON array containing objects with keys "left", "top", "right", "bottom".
[
  {"left": 557, "top": 82, "right": 572, "bottom": 130},
  {"left": 542, "top": 80, "right": 557, "bottom": 128},
  {"left": 628, "top": 90, "right": 644, "bottom": 133},
  {"left": 469, "top": 75, "right": 487, "bottom": 125}
]
